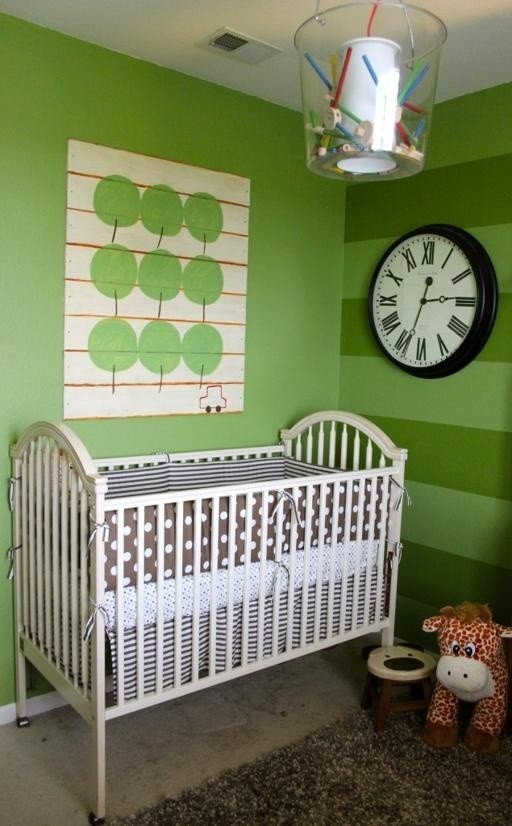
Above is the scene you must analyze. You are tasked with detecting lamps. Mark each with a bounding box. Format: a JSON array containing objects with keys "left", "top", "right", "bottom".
[{"left": 293, "top": 1, "right": 448, "bottom": 183}]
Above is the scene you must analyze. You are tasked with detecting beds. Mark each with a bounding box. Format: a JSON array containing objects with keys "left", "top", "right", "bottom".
[{"left": 9, "top": 411, "right": 409, "bottom": 825}]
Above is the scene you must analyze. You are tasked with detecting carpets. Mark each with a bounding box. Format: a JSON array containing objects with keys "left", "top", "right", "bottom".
[{"left": 107, "top": 709, "right": 511, "bottom": 825}]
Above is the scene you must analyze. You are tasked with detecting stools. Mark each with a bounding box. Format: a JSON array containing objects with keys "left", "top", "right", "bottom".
[{"left": 361, "top": 643, "right": 437, "bottom": 732}]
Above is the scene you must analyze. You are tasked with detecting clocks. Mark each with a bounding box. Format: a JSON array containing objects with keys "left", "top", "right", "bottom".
[{"left": 368, "top": 224, "right": 498, "bottom": 379}]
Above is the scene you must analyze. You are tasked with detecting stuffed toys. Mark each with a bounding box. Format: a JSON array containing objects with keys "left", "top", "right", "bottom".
[{"left": 422, "top": 602, "right": 512, "bottom": 754}]
